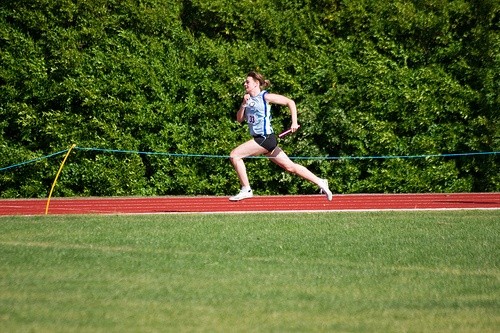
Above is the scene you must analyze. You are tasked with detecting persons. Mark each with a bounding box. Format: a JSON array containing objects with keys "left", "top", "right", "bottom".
[{"left": 229, "top": 71, "right": 332, "bottom": 201}]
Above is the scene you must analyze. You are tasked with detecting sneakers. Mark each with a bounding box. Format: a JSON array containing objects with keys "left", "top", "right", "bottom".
[
  {"left": 320, "top": 178, "right": 333, "bottom": 203},
  {"left": 228, "top": 186, "right": 253, "bottom": 201}
]
